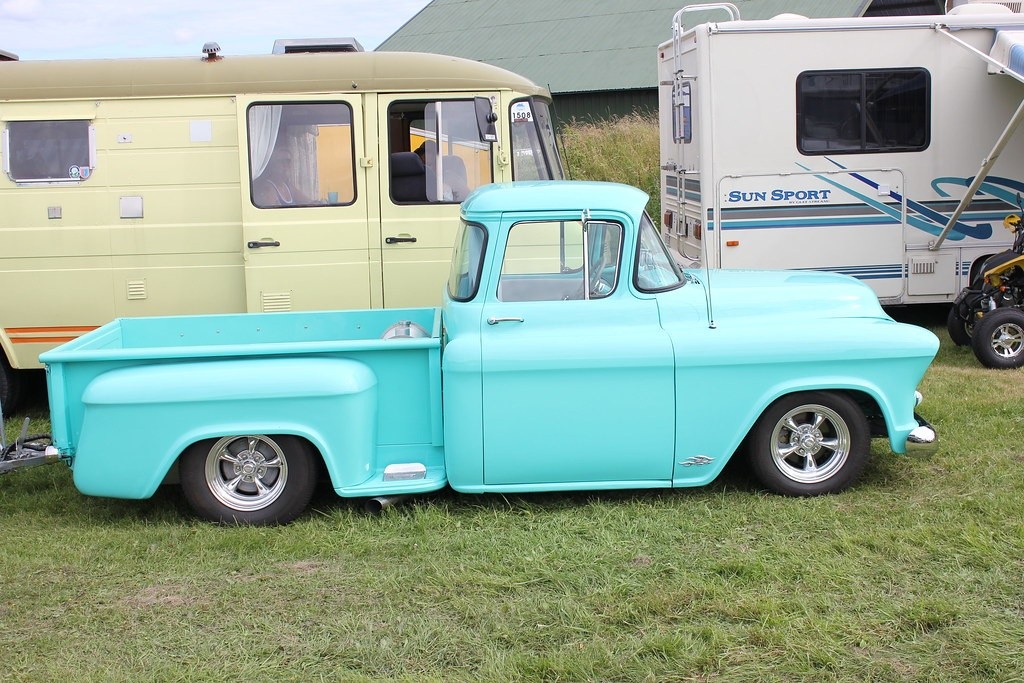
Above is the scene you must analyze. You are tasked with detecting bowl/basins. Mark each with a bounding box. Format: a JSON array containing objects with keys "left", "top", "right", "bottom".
[{"left": 381, "top": 320, "right": 431, "bottom": 338}]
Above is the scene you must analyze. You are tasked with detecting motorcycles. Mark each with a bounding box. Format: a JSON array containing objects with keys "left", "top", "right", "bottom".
[{"left": 945, "top": 192, "right": 1024, "bottom": 371}]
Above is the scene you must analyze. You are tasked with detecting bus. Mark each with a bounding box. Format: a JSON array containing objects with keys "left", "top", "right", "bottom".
[{"left": 0, "top": 48, "right": 581, "bottom": 413}]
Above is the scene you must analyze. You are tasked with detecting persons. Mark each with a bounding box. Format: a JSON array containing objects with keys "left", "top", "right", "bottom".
[
  {"left": 255, "top": 146, "right": 331, "bottom": 207},
  {"left": 414, "top": 146, "right": 471, "bottom": 204}
]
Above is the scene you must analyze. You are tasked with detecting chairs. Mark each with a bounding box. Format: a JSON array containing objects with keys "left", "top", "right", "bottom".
[{"left": 390, "top": 149, "right": 454, "bottom": 206}]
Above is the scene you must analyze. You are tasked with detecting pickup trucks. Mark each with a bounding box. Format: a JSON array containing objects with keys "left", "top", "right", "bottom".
[{"left": 35, "top": 180, "right": 942, "bottom": 526}]
